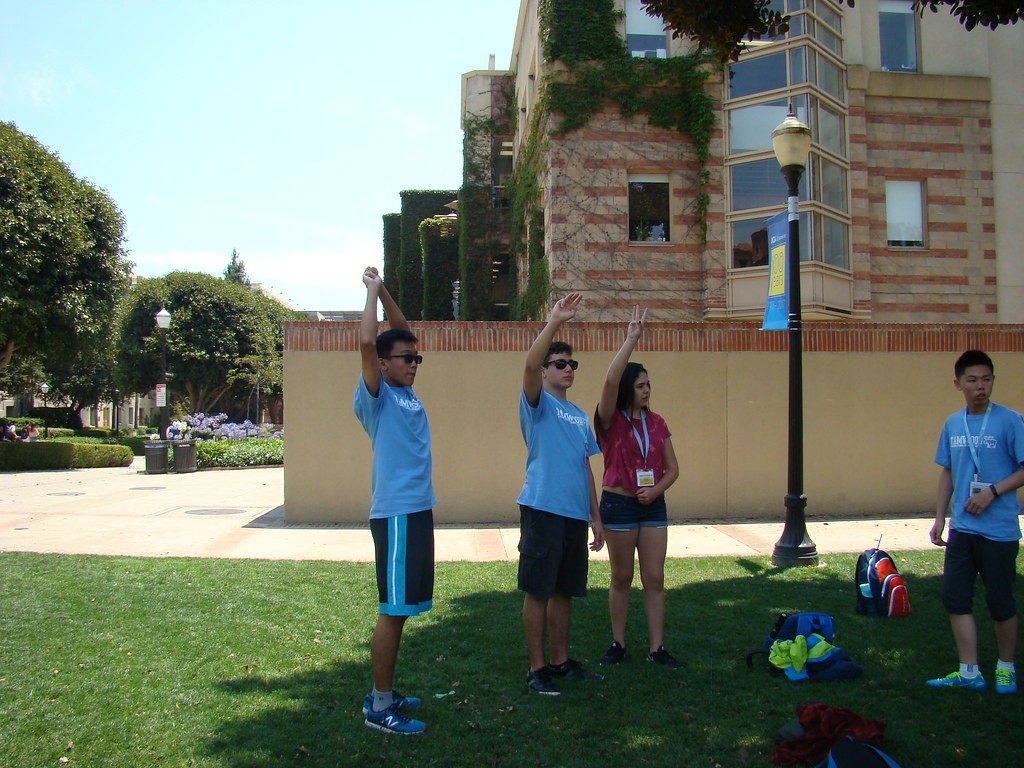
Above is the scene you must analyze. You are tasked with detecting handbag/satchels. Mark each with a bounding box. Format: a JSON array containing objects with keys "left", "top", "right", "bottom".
[
  {"left": 855, "top": 549, "right": 910, "bottom": 618},
  {"left": 747, "top": 612, "right": 835, "bottom": 671}
]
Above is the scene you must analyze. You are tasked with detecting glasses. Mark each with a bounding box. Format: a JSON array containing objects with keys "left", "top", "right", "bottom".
[
  {"left": 380, "top": 354, "right": 422, "bottom": 364},
  {"left": 544, "top": 359, "right": 579, "bottom": 370}
]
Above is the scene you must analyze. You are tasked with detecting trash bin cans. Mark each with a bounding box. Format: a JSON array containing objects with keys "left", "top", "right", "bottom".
[
  {"left": 172, "top": 439, "right": 197, "bottom": 473},
  {"left": 143, "top": 440, "right": 172, "bottom": 474}
]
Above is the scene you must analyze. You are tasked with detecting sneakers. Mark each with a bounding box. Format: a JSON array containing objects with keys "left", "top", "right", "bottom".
[
  {"left": 646, "top": 645, "right": 684, "bottom": 669},
  {"left": 600, "top": 641, "right": 627, "bottom": 665},
  {"left": 995, "top": 666, "right": 1017, "bottom": 694},
  {"left": 925, "top": 672, "right": 986, "bottom": 691},
  {"left": 526, "top": 665, "right": 562, "bottom": 695},
  {"left": 362, "top": 689, "right": 421, "bottom": 714},
  {"left": 365, "top": 703, "right": 429, "bottom": 734},
  {"left": 548, "top": 658, "right": 604, "bottom": 680}
]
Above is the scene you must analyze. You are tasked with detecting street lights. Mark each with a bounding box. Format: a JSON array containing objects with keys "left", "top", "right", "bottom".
[
  {"left": 41, "top": 382, "right": 49, "bottom": 437},
  {"left": 156, "top": 305, "right": 171, "bottom": 441},
  {"left": 771, "top": 102, "right": 819, "bottom": 564}
]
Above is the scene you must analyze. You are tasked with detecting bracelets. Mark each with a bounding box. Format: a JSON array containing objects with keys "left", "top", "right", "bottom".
[{"left": 989, "top": 484, "right": 998, "bottom": 497}]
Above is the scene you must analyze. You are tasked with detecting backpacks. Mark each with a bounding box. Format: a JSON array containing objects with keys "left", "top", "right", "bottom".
[{"left": 813, "top": 736, "right": 900, "bottom": 768}]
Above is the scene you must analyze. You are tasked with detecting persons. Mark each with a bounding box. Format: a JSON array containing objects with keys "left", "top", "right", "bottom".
[
  {"left": 353, "top": 266, "right": 438, "bottom": 735},
  {"left": 594, "top": 305, "right": 688, "bottom": 671},
  {"left": 925, "top": 351, "right": 1024, "bottom": 695},
  {"left": 516, "top": 292, "right": 605, "bottom": 697},
  {"left": 0, "top": 420, "right": 41, "bottom": 442}
]
